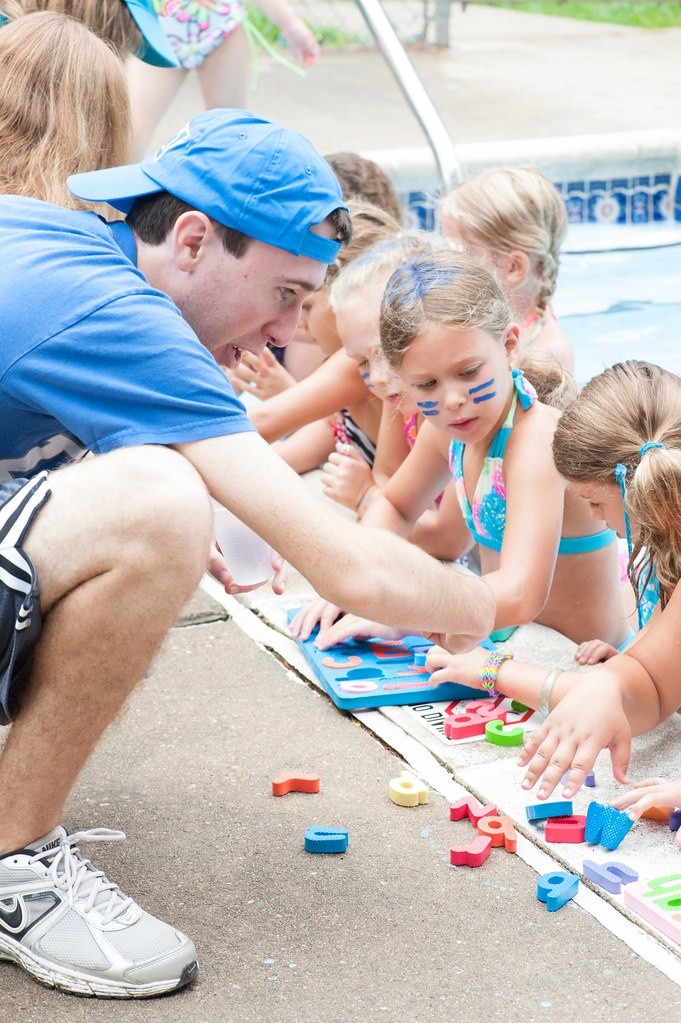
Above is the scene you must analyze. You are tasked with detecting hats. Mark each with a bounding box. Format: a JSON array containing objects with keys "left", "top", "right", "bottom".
[
  {"left": 67, "top": 106, "right": 351, "bottom": 264},
  {"left": 118, "top": 0, "right": 181, "bottom": 70}
]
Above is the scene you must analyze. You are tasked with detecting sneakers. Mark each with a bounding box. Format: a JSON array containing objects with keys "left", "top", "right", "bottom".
[{"left": 1, "top": 826, "right": 198, "bottom": 998}]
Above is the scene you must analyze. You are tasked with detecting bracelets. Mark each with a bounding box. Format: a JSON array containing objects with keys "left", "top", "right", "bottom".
[
  {"left": 479, "top": 649, "right": 513, "bottom": 698},
  {"left": 538, "top": 667, "right": 565, "bottom": 721}
]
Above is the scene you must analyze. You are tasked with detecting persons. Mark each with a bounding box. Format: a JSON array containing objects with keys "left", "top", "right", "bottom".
[
  {"left": 286, "top": 248, "right": 636, "bottom": 652},
  {"left": 0, "top": 108, "right": 497, "bottom": 1001},
  {"left": 0, "top": 1, "right": 180, "bottom": 81},
  {"left": 2, "top": 11, "right": 131, "bottom": 219},
  {"left": 515, "top": 575, "right": 681, "bottom": 823},
  {"left": 220, "top": 151, "right": 567, "bottom": 562},
  {"left": 422, "top": 357, "right": 680, "bottom": 718},
  {"left": 119, "top": 1, "right": 321, "bottom": 166}
]
